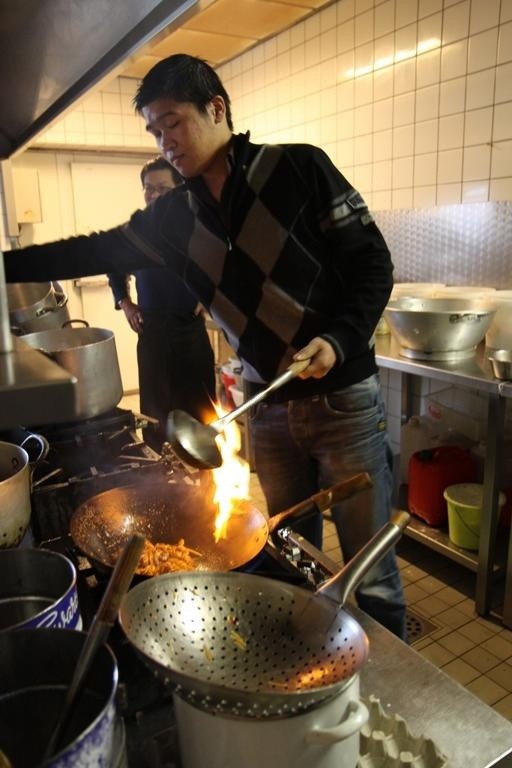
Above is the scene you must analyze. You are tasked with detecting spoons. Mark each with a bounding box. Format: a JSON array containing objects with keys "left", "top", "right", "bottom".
[{"left": 166, "top": 352, "right": 316, "bottom": 471}]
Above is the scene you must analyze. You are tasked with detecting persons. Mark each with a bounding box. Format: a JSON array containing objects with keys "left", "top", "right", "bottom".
[
  {"left": 105, "top": 155, "right": 217, "bottom": 457},
  {"left": 2, "top": 53, "right": 407, "bottom": 640}
]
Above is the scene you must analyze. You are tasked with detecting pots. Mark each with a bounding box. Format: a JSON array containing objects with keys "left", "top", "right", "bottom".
[
  {"left": 0, "top": 434, "right": 52, "bottom": 549},
  {"left": 9, "top": 282, "right": 69, "bottom": 321},
  {"left": 382, "top": 298, "right": 485, "bottom": 362},
  {"left": 67, "top": 474, "right": 374, "bottom": 577},
  {"left": 173, "top": 679, "right": 369, "bottom": 768},
  {"left": 15, "top": 319, "right": 124, "bottom": 424}
]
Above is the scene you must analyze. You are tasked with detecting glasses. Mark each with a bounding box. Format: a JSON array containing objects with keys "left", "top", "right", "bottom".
[{"left": 141, "top": 185, "right": 173, "bottom": 197}]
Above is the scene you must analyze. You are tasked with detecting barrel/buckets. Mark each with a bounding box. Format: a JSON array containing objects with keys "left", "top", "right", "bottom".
[
  {"left": 400, "top": 417, "right": 432, "bottom": 486},
  {"left": 444, "top": 484, "right": 505, "bottom": 550}
]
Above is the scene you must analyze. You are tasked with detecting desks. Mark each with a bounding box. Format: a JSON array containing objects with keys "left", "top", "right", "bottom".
[{"left": 360, "top": 324, "right": 508, "bottom": 617}]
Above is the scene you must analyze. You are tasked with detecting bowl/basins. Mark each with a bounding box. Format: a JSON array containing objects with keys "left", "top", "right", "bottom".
[{"left": 488, "top": 349, "right": 512, "bottom": 381}]
